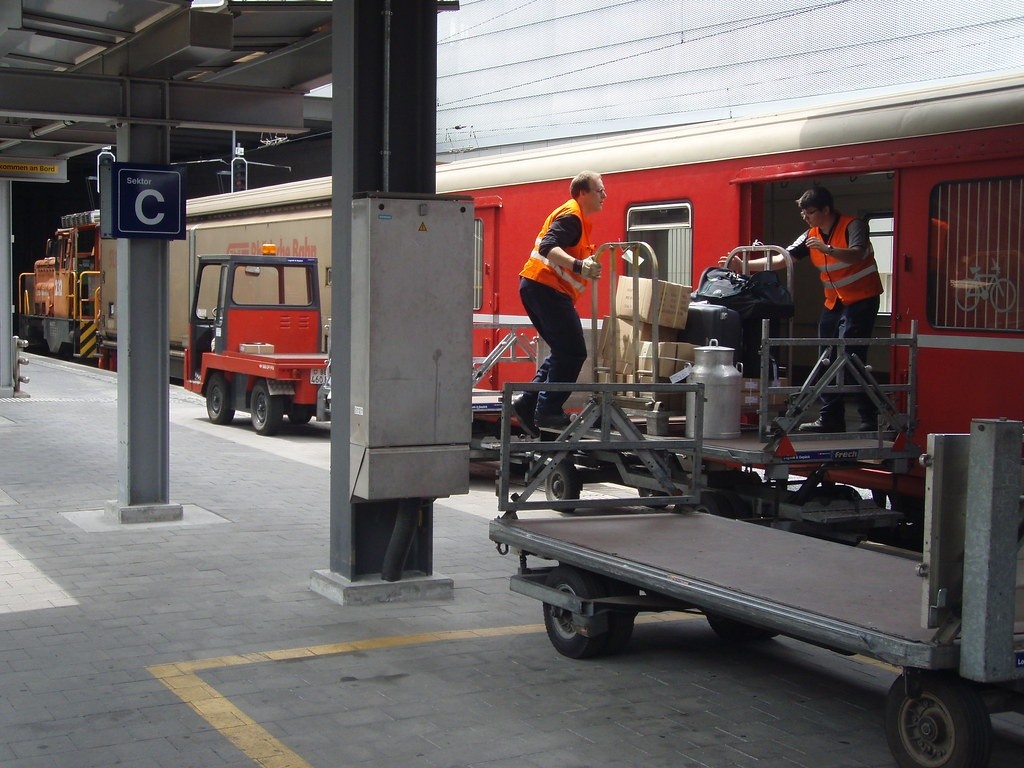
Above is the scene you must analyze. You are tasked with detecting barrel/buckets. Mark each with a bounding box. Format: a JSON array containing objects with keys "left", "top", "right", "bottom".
[{"left": 683, "top": 339, "right": 745, "bottom": 441}]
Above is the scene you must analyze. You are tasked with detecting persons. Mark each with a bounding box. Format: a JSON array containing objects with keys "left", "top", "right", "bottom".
[
  {"left": 509, "top": 170, "right": 606, "bottom": 438},
  {"left": 925, "top": 217, "right": 950, "bottom": 324},
  {"left": 717, "top": 186, "right": 884, "bottom": 433}
]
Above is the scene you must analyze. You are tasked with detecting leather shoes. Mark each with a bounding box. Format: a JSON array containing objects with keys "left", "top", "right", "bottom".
[
  {"left": 510, "top": 394, "right": 540, "bottom": 437},
  {"left": 799, "top": 415, "right": 847, "bottom": 432},
  {"left": 534, "top": 410, "right": 572, "bottom": 426},
  {"left": 860, "top": 419, "right": 877, "bottom": 431}
]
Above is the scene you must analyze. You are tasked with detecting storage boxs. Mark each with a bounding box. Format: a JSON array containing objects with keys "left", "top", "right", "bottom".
[{"left": 597, "top": 266, "right": 789, "bottom": 417}]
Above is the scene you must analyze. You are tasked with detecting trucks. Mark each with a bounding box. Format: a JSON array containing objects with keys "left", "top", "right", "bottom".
[{"left": 182, "top": 243, "right": 528, "bottom": 446}]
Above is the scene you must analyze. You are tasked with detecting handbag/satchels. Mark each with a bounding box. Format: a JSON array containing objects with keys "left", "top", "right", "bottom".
[{"left": 688, "top": 268, "right": 797, "bottom": 322}]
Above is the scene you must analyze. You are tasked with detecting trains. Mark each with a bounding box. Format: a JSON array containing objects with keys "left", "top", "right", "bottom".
[{"left": 16, "top": 68, "right": 1018, "bottom": 522}]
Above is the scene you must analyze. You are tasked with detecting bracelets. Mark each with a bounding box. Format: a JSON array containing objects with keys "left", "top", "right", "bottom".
[{"left": 824, "top": 245, "right": 833, "bottom": 256}]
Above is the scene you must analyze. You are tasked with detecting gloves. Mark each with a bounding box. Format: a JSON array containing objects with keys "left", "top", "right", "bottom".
[{"left": 573, "top": 254, "right": 601, "bottom": 281}]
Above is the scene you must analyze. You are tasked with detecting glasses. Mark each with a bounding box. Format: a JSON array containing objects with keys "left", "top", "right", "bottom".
[
  {"left": 589, "top": 186, "right": 606, "bottom": 195},
  {"left": 801, "top": 208, "right": 822, "bottom": 217}
]
